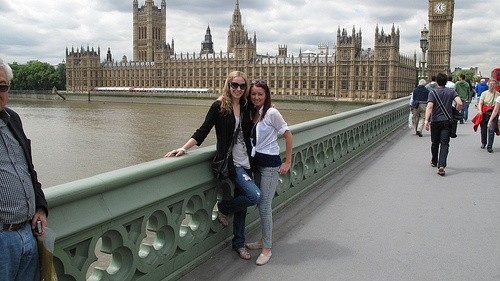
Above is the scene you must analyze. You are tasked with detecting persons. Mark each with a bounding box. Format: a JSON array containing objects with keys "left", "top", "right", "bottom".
[
  {"left": 486, "top": 95, "right": 500, "bottom": 130},
  {"left": 409, "top": 79, "right": 431, "bottom": 138},
  {"left": 424, "top": 74, "right": 463, "bottom": 175},
  {"left": 453, "top": 74, "right": 471, "bottom": 124},
  {"left": 444, "top": 74, "right": 457, "bottom": 108},
  {"left": 245, "top": 80, "right": 293, "bottom": 266},
  {"left": 163, "top": 70, "right": 262, "bottom": 260},
  {"left": 474, "top": 78, "right": 489, "bottom": 111},
  {"left": 464, "top": 79, "right": 474, "bottom": 109},
  {"left": 477, "top": 79, "right": 500, "bottom": 153},
  {"left": 425, "top": 76, "right": 438, "bottom": 93},
  {"left": 0, "top": 59, "right": 49, "bottom": 281}
]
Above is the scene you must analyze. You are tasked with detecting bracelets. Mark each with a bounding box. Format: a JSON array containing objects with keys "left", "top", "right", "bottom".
[{"left": 179, "top": 148, "right": 187, "bottom": 153}]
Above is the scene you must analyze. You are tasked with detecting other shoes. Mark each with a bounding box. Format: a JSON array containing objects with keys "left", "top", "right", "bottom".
[
  {"left": 256, "top": 250, "right": 273, "bottom": 265},
  {"left": 246, "top": 242, "right": 263, "bottom": 250},
  {"left": 416, "top": 131, "right": 423, "bottom": 136},
  {"left": 487, "top": 144, "right": 492, "bottom": 152},
  {"left": 479, "top": 141, "right": 486, "bottom": 148},
  {"left": 437, "top": 167, "right": 446, "bottom": 175},
  {"left": 431, "top": 158, "right": 436, "bottom": 166},
  {"left": 460, "top": 120, "right": 465, "bottom": 124},
  {"left": 218, "top": 203, "right": 228, "bottom": 226},
  {"left": 235, "top": 246, "right": 251, "bottom": 259}
]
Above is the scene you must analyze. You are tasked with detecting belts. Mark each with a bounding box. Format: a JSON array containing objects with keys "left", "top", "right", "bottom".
[{"left": 0, "top": 220, "right": 29, "bottom": 232}]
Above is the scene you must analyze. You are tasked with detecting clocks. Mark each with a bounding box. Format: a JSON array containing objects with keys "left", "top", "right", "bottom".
[{"left": 433, "top": 2, "right": 446, "bottom": 14}]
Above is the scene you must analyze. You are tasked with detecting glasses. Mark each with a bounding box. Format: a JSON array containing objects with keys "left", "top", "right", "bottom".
[
  {"left": 250, "top": 79, "right": 268, "bottom": 85},
  {"left": 0, "top": 82, "right": 11, "bottom": 92},
  {"left": 230, "top": 82, "right": 247, "bottom": 90}
]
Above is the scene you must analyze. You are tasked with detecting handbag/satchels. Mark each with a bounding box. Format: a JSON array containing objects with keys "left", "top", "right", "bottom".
[
  {"left": 212, "top": 153, "right": 236, "bottom": 180},
  {"left": 37, "top": 225, "right": 57, "bottom": 281},
  {"left": 450, "top": 107, "right": 463, "bottom": 137}
]
